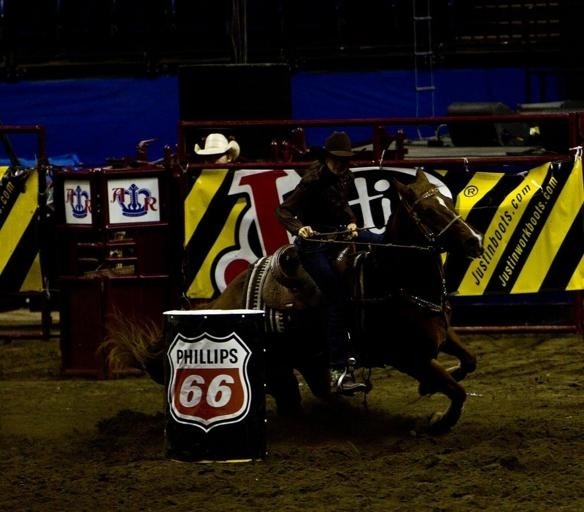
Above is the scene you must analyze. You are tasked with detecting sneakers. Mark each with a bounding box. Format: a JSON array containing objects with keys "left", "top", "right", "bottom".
[{"left": 328, "top": 367, "right": 367, "bottom": 394}]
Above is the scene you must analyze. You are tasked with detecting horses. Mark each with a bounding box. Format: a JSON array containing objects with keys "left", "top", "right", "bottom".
[{"left": 97, "top": 167, "right": 486, "bottom": 433}]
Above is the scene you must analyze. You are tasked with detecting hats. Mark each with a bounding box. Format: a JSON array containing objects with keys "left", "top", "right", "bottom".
[{"left": 321, "top": 131, "right": 367, "bottom": 158}]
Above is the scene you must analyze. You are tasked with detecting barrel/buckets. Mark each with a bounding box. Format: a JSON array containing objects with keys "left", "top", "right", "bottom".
[{"left": 163, "top": 309, "right": 264, "bottom": 461}]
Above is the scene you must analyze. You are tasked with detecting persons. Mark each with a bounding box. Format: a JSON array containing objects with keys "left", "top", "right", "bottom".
[{"left": 272, "top": 129, "right": 368, "bottom": 396}]
